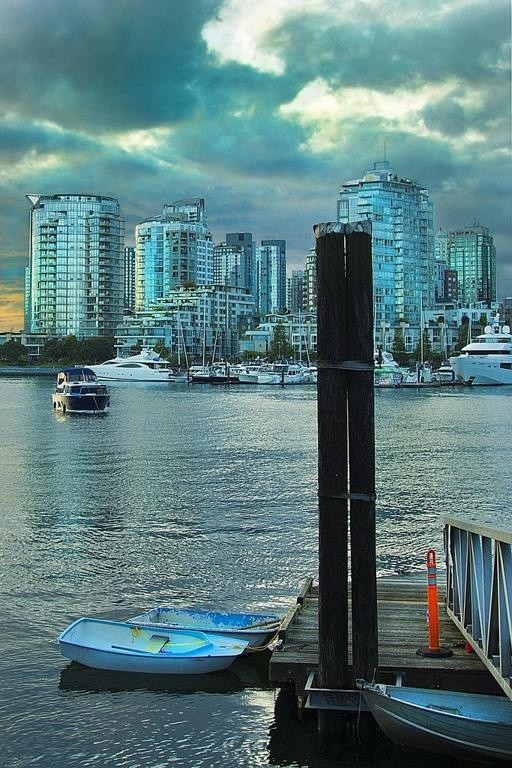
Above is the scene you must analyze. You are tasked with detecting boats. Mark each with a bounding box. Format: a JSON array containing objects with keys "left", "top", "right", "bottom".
[
  {"left": 449, "top": 311, "right": 512, "bottom": 386},
  {"left": 169, "top": 293, "right": 454, "bottom": 385},
  {"left": 58, "top": 618, "right": 247, "bottom": 675},
  {"left": 53, "top": 369, "right": 110, "bottom": 413},
  {"left": 355, "top": 681, "right": 511, "bottom": 768},
  {"left": 87, "top": 344, "right": 171, "bottom": 383},
  {"left": 128, "top": 608, "right": 279, "bottom": 657}
]
